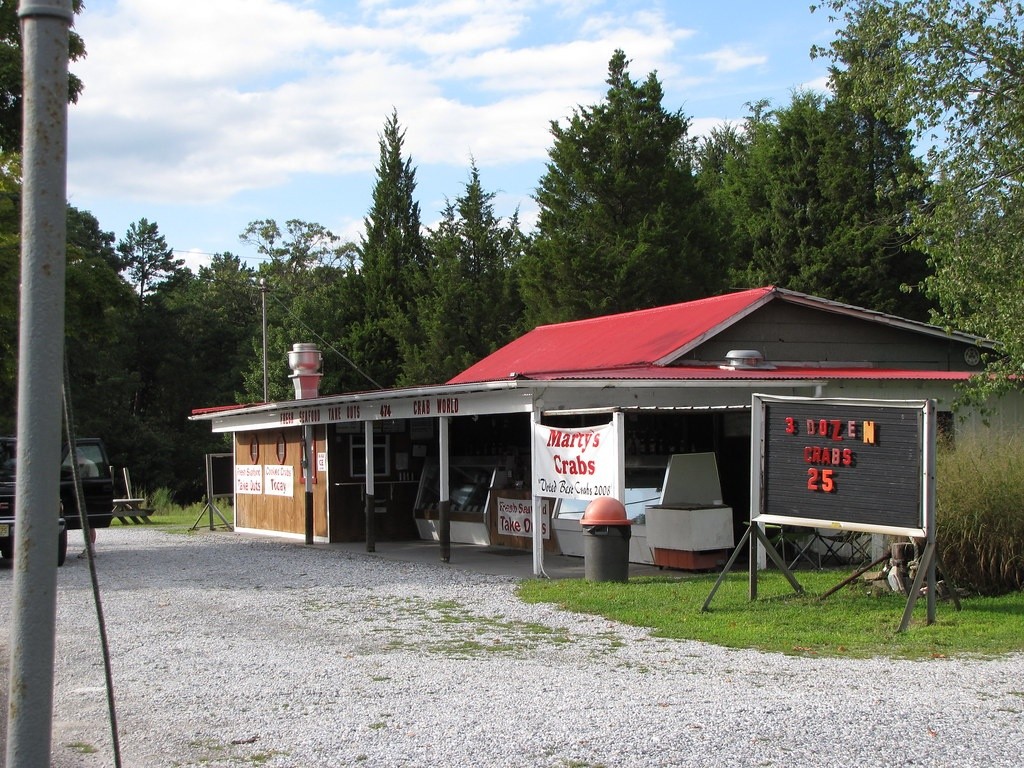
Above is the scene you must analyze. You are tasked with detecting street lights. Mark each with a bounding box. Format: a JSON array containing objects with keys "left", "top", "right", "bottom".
[{"left": 259, "top": 278, "right": 267, "bottom": 402}]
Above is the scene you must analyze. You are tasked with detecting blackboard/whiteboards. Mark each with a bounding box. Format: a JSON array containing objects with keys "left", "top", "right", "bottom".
[{"left": 206, "top": 453, "right": 234, "bottom": 497}]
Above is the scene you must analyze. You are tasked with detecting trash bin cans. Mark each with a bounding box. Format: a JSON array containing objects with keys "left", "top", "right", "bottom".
[{"left": 581, "top": 497, "right": 633, "bottom": 583}]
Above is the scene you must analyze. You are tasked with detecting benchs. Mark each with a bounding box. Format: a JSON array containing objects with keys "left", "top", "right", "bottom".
[{"left": 112, "top": 507, "right": 156, "bottom": 524}]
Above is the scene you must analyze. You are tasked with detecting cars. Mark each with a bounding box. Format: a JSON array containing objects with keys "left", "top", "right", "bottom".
[{"left": 0, "top": 436, "right": 113, "bottom": 567}]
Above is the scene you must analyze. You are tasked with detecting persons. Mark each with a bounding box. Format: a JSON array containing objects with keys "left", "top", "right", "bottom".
[{"left": 67, "top": 447, "right": 99, "bottom": 559}]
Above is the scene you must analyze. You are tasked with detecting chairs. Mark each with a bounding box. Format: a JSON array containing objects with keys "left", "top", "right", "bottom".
[{"left": 742, "top": 522, "right": 874, "bottom": 570}]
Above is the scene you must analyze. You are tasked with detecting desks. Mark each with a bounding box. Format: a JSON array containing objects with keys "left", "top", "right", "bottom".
[{"left": 113, "top": 498, "right": 153, "bottom": 524}]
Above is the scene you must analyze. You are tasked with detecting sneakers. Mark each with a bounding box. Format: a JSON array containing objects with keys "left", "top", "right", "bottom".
[{"left": 77, "top": 549, "right": 97, "bottom": 558}]
